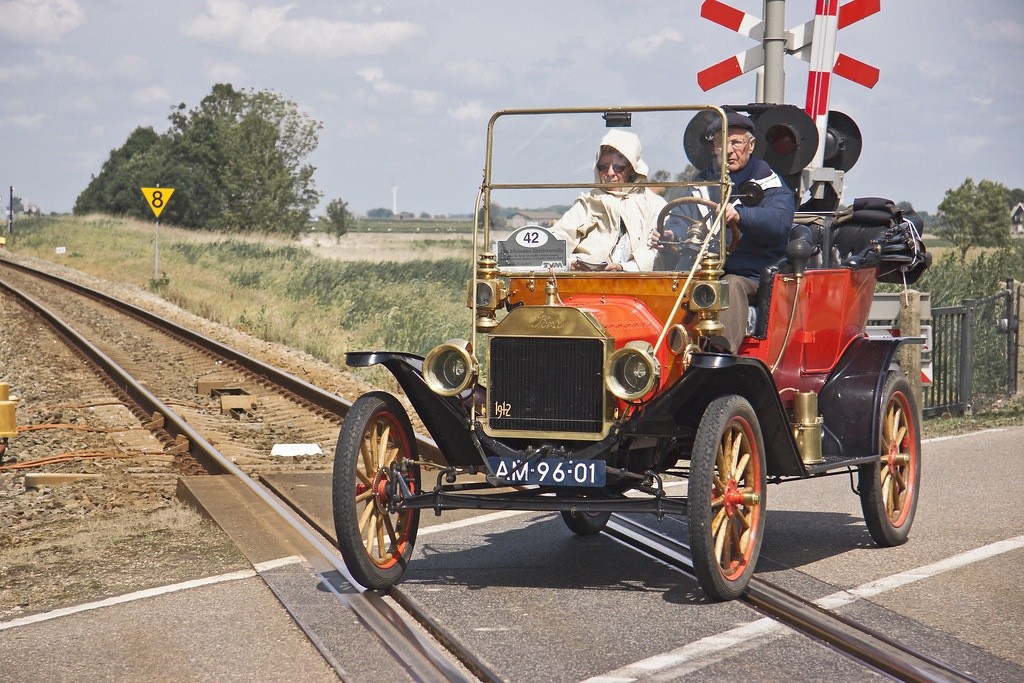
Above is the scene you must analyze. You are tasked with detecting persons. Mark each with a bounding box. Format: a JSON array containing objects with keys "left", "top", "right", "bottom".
[
  {"left": 546, "top": 128, "right": 671, "bottom": 272},
  {"left": 650, "top": 113, "right": 796, "bottom": 356}
]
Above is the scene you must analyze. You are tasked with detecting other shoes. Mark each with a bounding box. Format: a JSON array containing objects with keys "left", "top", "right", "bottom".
[{"left": 704, "top": 345, "right": 731, "bottom": 354}]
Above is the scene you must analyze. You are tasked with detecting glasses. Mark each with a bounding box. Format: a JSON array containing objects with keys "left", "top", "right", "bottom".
[
  {"left": 596, "top": 163, "right": 631, "bottom": 173},
  {"left": 715, "top": 137, "right": 754, "bottom": 149}
]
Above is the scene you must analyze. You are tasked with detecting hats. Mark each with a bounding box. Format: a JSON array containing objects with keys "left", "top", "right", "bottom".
[{"left": 705, "top": 113, "right": 754, "bottom": 143}]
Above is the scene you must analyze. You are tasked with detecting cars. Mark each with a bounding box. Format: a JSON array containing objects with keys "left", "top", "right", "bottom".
[{"left": 331, "top": 106, "right": 933, "bottom": 603}]
